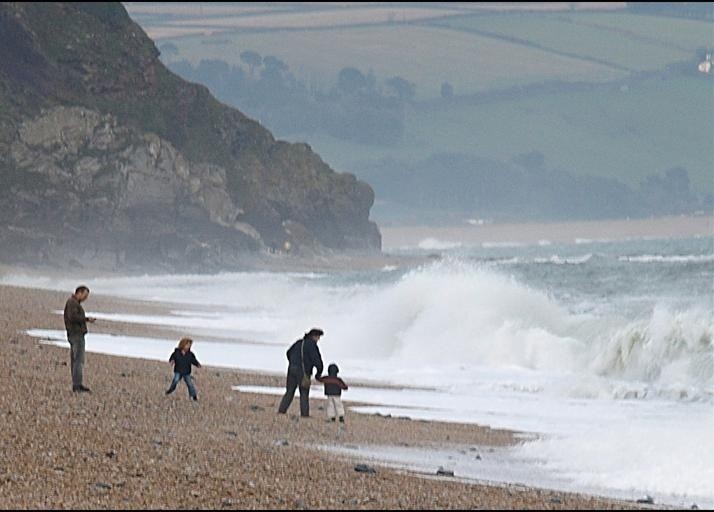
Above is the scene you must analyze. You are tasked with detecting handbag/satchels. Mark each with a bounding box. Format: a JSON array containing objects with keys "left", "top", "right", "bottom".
[{"left": 301, "top": 376, "right": 311, "bottom": 388}]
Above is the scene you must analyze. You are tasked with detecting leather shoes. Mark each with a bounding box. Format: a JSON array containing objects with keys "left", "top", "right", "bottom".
[{"left": 73, "top": 385, "right": 89, "bottom": 391}]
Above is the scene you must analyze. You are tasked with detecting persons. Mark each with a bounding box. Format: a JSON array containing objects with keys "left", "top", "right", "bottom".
[
  {"left": 63, "top": 286, "right": 97, "bottom": 392},
  {"left": 164, "top": 336, "right": 199, "bottom": 401},
  {"left": 315, "top": 364, "right": 349, "bottom": 423},
  {"left": 276, "top": 328, "right": 322, "bottom": 418}
]
